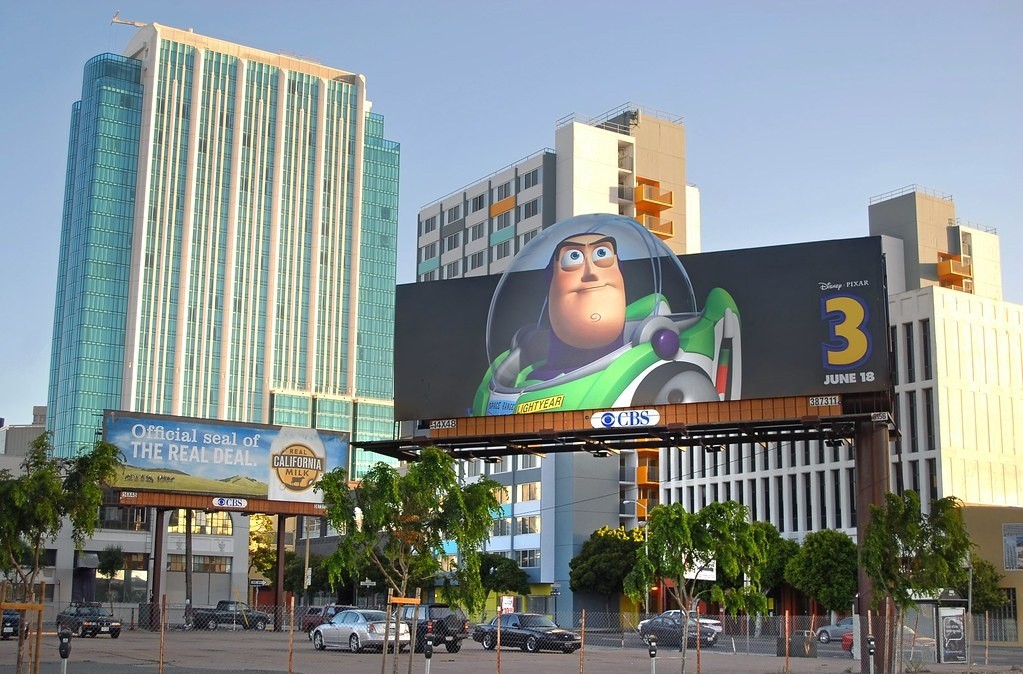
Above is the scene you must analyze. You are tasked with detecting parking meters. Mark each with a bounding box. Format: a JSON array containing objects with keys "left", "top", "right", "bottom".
[
  {"left": 647, "top": 635, "right": 658, "bottom": 674},
  {"left": 423, "top": 633, "right": 435, "bottom": 674},
  {"left": 58, "top": 629, "right": 72, "bottom": 674},
  {"left": 866, "top": 634, "right": 876, "bottom": 674}
]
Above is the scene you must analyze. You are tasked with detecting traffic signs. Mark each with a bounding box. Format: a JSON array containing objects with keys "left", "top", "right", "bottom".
[{"left": 249, "top": 579, "right": 266, "bottom": 586}]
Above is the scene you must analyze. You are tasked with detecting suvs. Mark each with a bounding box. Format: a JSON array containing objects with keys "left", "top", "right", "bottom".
[
  {"left": 392, "top": 603, "right": 470, "bottom": 653},
  {"left": 56, "top": 601, "right": 121, "bottom": 639}
]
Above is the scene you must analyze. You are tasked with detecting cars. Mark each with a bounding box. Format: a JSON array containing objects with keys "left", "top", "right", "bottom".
[
  {"left": 312, "top": 610, "right": 411, "bottom": 653},
  {"left": 638, "top": 610, "right": 722, "bottom": 647},
  {"left": 1, "top": 608, "right": 29, "bottom": 641},
  {"left": 816, "top": 613, "right": 935, "bottom": 655},
  {"left": 472, "top": 613, "right": 581, "bottom": 654}
]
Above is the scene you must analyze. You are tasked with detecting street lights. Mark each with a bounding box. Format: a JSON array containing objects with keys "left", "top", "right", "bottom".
[{"left": 623, "top": 500, "right": 649, "bottom": 618}]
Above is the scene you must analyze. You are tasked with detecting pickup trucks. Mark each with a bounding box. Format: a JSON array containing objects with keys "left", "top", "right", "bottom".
[
  {"left": 193, "top": 599, "right": 271, "bottom": 631},
  {"left": 302, "top": 604, "right": 359, "bottom": 641}
]
